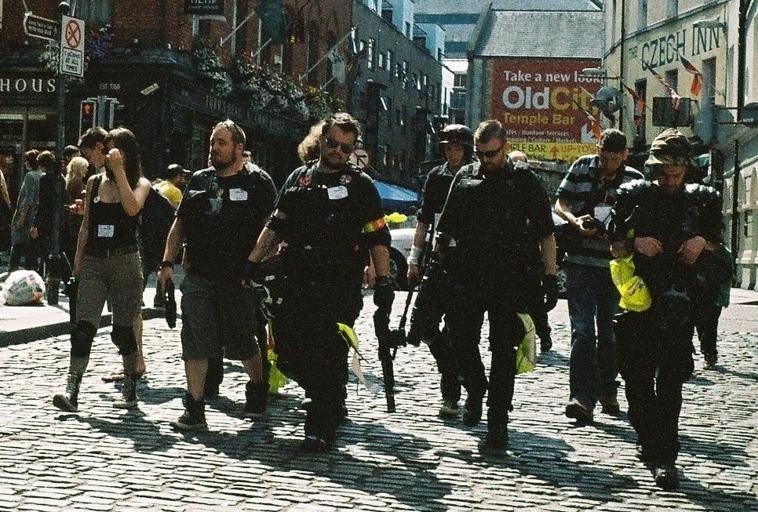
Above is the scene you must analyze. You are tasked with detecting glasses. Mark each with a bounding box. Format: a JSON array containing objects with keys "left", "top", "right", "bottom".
[
  {"left": 321, "top": 134, "right": 358, "bottom": 156},
  {"left": 472, "top": 142, "right": 509, "bottom": 158},
  {"left": 99, "top": 148, "right": 123, "bottom": 154}
]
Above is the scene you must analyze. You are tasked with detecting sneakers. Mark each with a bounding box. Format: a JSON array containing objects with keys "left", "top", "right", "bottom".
[
  {"left": 485, "top": 408, "right": 509, "bottom": 446},
  {"left": 704, "top": 354, "right": 721, "bottom": 371},
  {"left": 438, "top": 400, "right": 488, "bottom": 426},
  {"left": 538, "top": 326, "right": 552, "bottom": 353},
  {"left": 565, "top": 395, "right": 595, "bottom": 423},
  {"left": 600, "top": 382, "right": 623, "bottom": 413},
  {"left": 170, "top": 377, "right": 224, "bottom": 429},
  {"left": 243, "top": 379, "right": 271, "bottom": 419},
  {"left": 303, "top": 407, "right": 347, "bottom": 452},
  {"left": 635, "top": 427, "right": 678, "bottom": 491}
]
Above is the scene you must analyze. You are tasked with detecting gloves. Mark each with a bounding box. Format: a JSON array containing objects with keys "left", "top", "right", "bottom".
[
  {"left": 372, "top": 275, "right": 394, "bottom": 312},
  {"left": 538, "top": 272, "right": 560, "bottom": 312},
  {"left": 240, "top": 260, "right": 257, "bottom": 288}
]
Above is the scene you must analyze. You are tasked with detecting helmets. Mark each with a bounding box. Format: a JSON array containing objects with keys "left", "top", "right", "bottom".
[{"left": 439, "top": 123, "right": 473, "bottom": 163}]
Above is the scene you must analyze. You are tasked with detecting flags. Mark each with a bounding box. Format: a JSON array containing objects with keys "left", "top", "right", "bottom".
[
  {"left": 328, "top": 43, "right": 345, "bottom": 86},
  {"left": 254, "top": 1, "right": 287, "bottom": 47},
  {"left": 619, "top": 77, "right": 646, "bottom": 123},
  {"left": 345, "top": 56, "right": 362, "bottom": 90},
  {"left": 645, "top": 61, "right": 681, "bottom": 111},
  {"left": 679, "top": 51, "right": 706, "bottom": 97},
  {"left": 578, "top": 105, "right": 601, "bottom": 138}
]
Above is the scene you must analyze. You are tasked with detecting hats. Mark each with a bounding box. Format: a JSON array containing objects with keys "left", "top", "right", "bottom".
[{"left": 644, "top": 128, "right": 696, "bottom": 168}]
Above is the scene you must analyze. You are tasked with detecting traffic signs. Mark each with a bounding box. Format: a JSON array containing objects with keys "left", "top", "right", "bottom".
[{"left": 24, "top": 15, "right": 61, "bottom": 41}]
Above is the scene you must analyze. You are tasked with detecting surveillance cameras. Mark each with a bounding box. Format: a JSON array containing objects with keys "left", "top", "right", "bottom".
[{"left": 59, "top": 2, "right": 70, "bottom": 13}]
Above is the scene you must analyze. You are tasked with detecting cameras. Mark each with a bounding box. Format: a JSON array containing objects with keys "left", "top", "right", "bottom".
[{"left": 582, "top": 217, "right": 606, "bottom": 235}]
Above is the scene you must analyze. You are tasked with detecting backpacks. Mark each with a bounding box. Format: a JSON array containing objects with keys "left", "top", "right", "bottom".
[{"left": 139, "top": 187, "right": 177, "bottom": 262}]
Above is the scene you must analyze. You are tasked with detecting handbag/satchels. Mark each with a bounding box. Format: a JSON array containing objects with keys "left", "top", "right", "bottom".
[{"left": 550, "top": 208, "right": 575, "bottom": 268}]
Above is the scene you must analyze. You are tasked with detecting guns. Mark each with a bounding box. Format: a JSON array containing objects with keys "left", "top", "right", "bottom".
[
  {"left": 391, "top": 224, "right": 434, "bottom": 359},
  {"left": 373, "top": 288, "right": 404, "bottom": 413},
  {"left": 62, "top": 277, "right": 80, "bottom": 321},
  {"left": 157, "top": 271, "right": 176, "bottom": 327}
]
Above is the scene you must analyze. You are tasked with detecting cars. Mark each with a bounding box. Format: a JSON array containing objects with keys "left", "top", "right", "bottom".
[{"left": 386, "top": 211, "right": 570, "bottom": 299}]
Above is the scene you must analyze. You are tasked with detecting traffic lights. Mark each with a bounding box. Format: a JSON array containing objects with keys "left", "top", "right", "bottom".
[
  {"left": 81, "top": 103, "right": 93, "bottom": 132},
  {"left": 115, "top": 104, "right": 127, "bottom": 130}
]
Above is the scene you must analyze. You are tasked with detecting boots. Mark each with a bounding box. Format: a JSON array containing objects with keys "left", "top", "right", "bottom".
[
  {"left": 101, "top": 366, "right": 146, "bottom": 408},
  {"left": 51, "top": 372, "right": 81, "bottom": 411}
]
